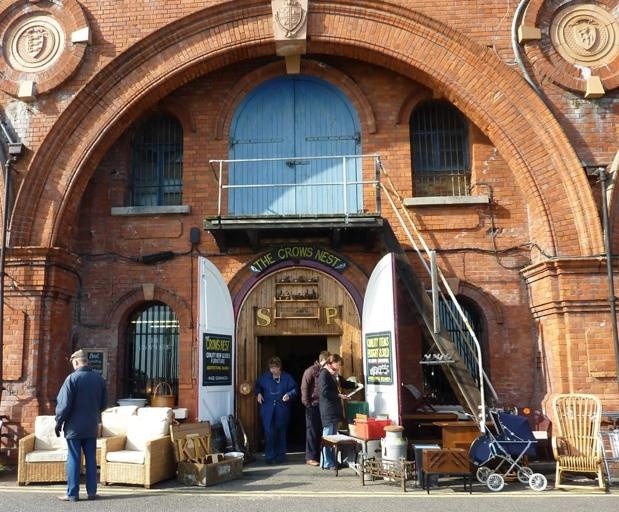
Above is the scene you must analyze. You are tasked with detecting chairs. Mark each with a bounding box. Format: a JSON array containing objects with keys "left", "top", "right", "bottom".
[
  {"left": 95, "top": 404, "right": 137, "bottom": 466},
  {"left": 100, "top": 415, "right": 177, "bottom": 492},
  {"left": 548, "top": 392, "right": 609, "bottom": 492},
  {"left": 17, "top": 413, "right": 72, "bottom": 488}
]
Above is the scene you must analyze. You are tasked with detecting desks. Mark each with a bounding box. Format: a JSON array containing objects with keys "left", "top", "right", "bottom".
[{"left": 318, "top": 406, "right": 532, "bottom": 493}]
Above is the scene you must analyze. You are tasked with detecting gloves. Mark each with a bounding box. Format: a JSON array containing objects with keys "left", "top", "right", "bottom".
[{"left": 54, "top": 425, "right": 62, "bottom": 438}]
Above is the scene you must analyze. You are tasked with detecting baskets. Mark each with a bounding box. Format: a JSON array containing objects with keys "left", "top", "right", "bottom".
[{"left": 149, "top": 382, "right": 177, "bottom": 408}]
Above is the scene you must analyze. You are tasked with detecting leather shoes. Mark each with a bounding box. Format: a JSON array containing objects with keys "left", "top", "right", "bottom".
[
  {"left": 305, "top": 459, "right": 320, "bottom": 466},
  {"left": 56, "top": 494, "right": 79, "bottom": 502},
  {"left": 88, "top": 496, "right": 96, "bottom": 500}
]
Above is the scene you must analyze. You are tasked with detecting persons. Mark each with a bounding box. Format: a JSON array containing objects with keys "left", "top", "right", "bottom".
[
  {"left": 301, "top": 350, "right": 332, "bottom": 466},
  {"left": 254, "top": 355, "right": 297, "bottom": 465},
  {"left": 55, "top": 349, "right": 107, "bottom": 502},
  {"left": 318, "top": 354, "right": 362, "bottom": 470}
]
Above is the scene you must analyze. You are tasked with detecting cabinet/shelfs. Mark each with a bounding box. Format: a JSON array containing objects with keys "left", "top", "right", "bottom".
[{"left": 272, "top": 276, "right": 322, "bottom": 321}]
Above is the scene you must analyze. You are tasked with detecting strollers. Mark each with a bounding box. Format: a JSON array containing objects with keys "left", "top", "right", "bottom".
[{"left": 461, "top": 408, "right": 549, "bottom": 494}]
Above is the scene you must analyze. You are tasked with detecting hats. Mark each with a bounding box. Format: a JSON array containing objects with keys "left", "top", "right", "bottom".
[{"left": 69, "top": 348, "right": 89, "bottom": 362}]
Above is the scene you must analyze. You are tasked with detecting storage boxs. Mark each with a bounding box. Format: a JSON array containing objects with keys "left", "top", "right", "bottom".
[{"left": 170, "top": 420, "right": 244, "bottom": 489}]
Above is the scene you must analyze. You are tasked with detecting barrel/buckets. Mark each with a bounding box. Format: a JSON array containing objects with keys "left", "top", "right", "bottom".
[
  {"left": 345, "top": 400, "right": 367, "bottom": 423},
  {"left": 117, "top": 399, "right": 147, "bottom": 407},
  {"left": 150, "top": 382, "right": 175, "bottom": 407}
]
